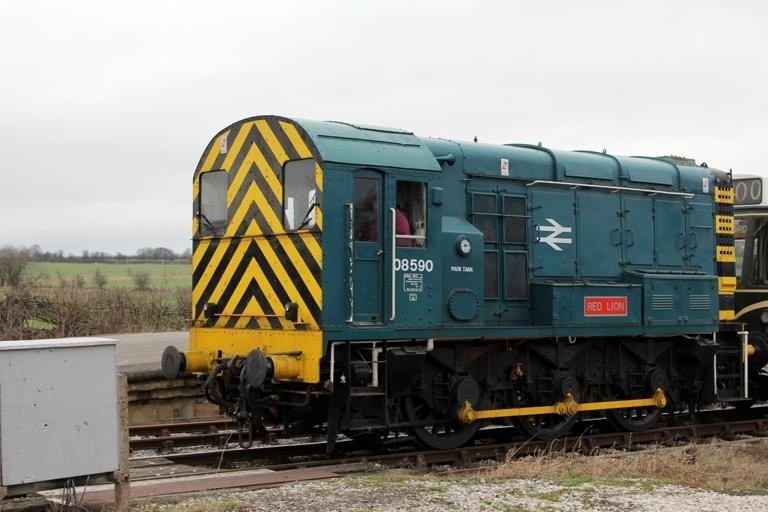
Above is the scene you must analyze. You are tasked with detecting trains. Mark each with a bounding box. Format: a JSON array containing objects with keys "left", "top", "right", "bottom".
[{"left": 163, "top": 115, "right": 764, "bottom": 449}]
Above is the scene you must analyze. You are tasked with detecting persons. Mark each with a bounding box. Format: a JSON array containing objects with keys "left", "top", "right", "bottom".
[{"left": 370, "top": 194, "right": 412, "bottom": 247}]
